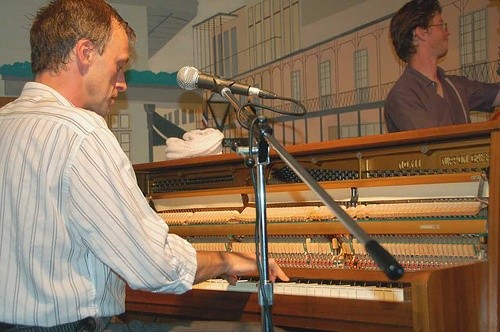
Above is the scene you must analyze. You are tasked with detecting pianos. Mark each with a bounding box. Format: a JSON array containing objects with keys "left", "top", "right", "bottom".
[{"left": 126, "top": 122, "right": 499, "bottom": 331}]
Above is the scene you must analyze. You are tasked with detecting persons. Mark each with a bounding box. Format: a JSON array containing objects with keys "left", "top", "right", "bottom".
[
  {"left": 0, "top": 0, "right": 288, "bottom": 332},
  {"left": 384, "top": 0, "right": 500, "bottom": 132}
]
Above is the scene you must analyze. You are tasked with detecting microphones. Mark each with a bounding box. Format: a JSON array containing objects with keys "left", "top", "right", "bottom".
[{"left": 176, "top": 66, "right": 277, "bottom": 102}]
